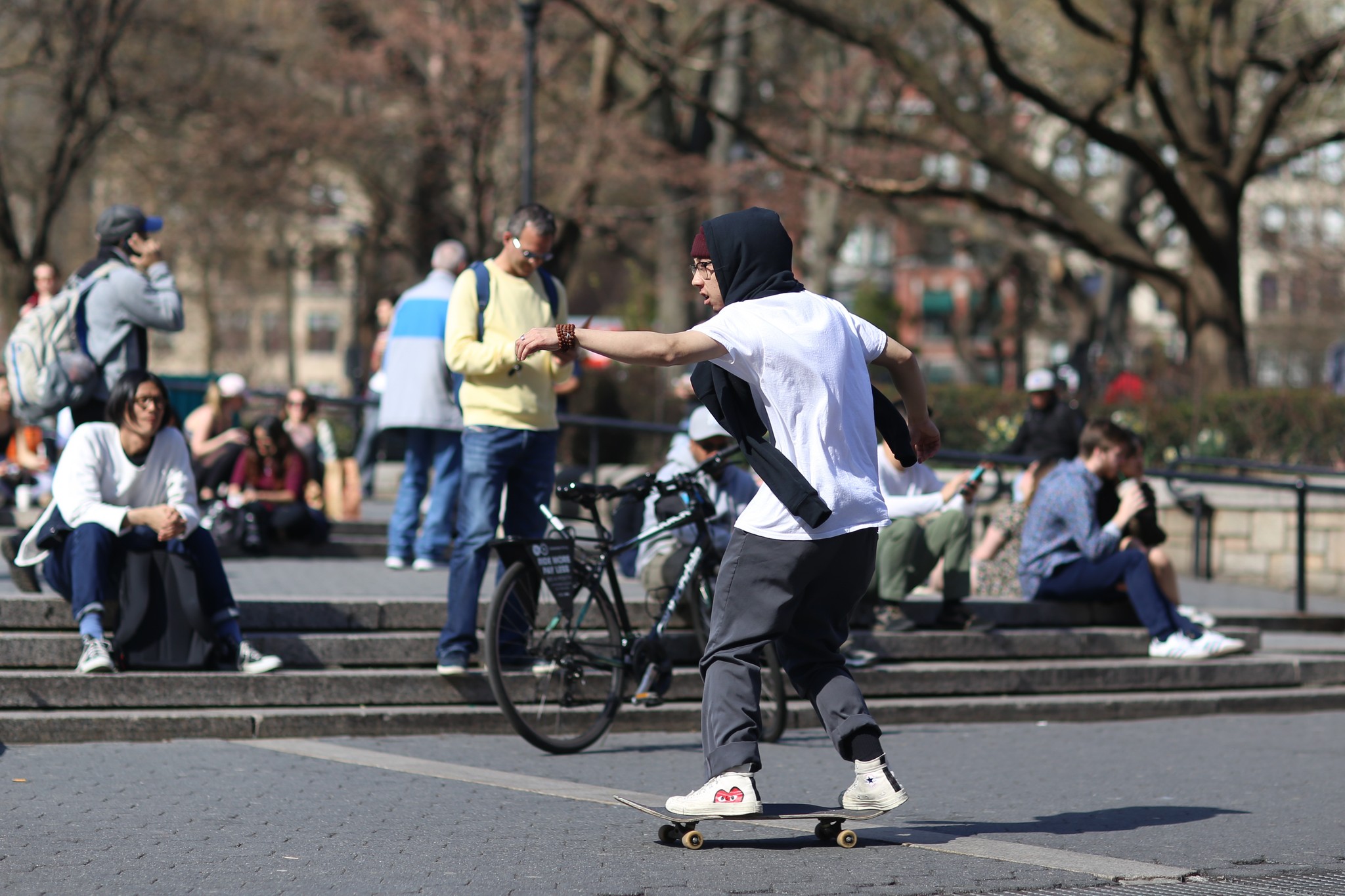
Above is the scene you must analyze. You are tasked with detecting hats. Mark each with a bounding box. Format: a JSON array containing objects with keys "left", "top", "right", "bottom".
[
  {"left": 1024, "top": 369, "right": 1053, "bottom": 393},
  {"left": 94, "top": 205, "right": 163, "bottom": 244},
  {"left": 690, "top": 226, "right": 709, "bottom": 256},
  {"left": 217, "top": 372, "right": 246, "bottom": 399},
  {"left": 688, "top": 404, "right": 733, "bottom": 443}
]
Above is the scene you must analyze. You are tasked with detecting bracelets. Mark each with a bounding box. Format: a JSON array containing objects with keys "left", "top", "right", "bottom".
[{"left": 556, "top": 324, "right": 576, "bottom": 354}]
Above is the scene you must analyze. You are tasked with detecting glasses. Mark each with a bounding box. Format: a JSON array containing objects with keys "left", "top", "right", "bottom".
[
  {"left": 690, "top": 261, "right": 712, "bottom": 279},
  {"left": 695, "top": 440, "right": 729, "bottom": 452},
  {"left": 134, "top": 395, "right": 165, "bottom": 410},
  {"left": 512, "top": 236, "right": 553, "bottom": 261}
]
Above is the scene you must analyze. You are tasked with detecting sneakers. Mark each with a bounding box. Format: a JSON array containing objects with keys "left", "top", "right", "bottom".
[
  {"left": 77, "top": 631, "right": 116, "bottom": 672},
  {"left": 838, "top": 755, "right": 909, "bottom": 811},
  {"left": 665, "top": 769, "right": 763, "bottom": 817},
  {"left": 226, "top": 640, "right": 282, "bottom": 673}
]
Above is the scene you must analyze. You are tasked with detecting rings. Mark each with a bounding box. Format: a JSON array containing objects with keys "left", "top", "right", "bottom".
[{"left": 521, "top": 335, "right": 525, "bottom": 341}]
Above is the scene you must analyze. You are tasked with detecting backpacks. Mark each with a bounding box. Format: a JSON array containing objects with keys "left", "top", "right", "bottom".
[{"left": 7, "top": 259, "right": 135, "bottom": 422}]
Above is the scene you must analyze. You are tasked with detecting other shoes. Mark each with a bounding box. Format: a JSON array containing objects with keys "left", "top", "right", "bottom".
[
  {"left": 385, "top": 555, "right": 405, "bottom": 569},
  {"left": 414, "top": 558, "right": 434, "bottom": 570},
  {"left": 875, "top": 604, "right": 914, "bottom": 632},
  {"left": 839, "top": 642, "right": 879, "bottom": 668},
  {"left": 436, "top": 650, "right": 466, "bottom": 674},
  {"left": 1201, "top": 629, "right": 1245, "bottom": 656},
  {"left": 484, "top": 651, "right": 558, "bottom": 674},
  {"left": 1177, "top": 604, "right": 1216, "bottom": 627},
  {"left": 934, "top": 606, "right": 994, "bottom": 631},
  {"left": 1146, "top": 631, "right": 1213, "bottom": 660}
]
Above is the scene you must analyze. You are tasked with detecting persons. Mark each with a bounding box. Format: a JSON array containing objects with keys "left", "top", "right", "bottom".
[
  {"left": 180, "top": 373, "right": 251, "bottom": 509},
  {"left": 515, "top": 206, "right": 941, "bottom": 815},
  {"left": 283, "top": 388, "right": 360, "bottom": 522},
  {"left": 1093, "top": 429, "right": 1216, "bottom": 629},
  {"left": 970, "top": 368, "right": 1089, "bottom": 598},
  {"left": 0, "top": 373, "right": 61, "bottom": 529},
  {"left": 434, "top": 204, "right": 576, "bottom": 674},
  {"left": 1019, "top": 417, "right": 1247, "bottom": 658},
  {"left": 4, "top": 205, "right": 185, "bottom": 450},
  {"left": 11, "top": 367, "right": 282, "bottom": 673},
  {"left": 633, "top": 402, "right": 876, "bottom": 667},
  {"left": 19, "top": 259, "right": 62, "bottom": 318},
  {"left": 852, "top": 400, "right": 998, "bottom": 630},
  {"left": 200, "top": 416, "right": 334, "bottom": 556},
  {"left": 353, "top": 295, "right": 397, "bottom": 498},
  {"left": 376, "top": 236, "right": 466, "bottom": 571}
]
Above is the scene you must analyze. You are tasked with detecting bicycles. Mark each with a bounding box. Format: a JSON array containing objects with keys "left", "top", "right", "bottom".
[{"left": 484, "top": 438, "right": 788, "bottom": 754}]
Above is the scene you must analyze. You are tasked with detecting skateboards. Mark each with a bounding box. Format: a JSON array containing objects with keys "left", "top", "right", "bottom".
[{"left": 613, "top": 795, "right": 892, "bottom": 850}]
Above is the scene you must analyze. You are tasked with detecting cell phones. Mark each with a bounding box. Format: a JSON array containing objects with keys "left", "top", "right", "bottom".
[
  {"left": 122, "top": 237, "right": 140, "bottom": 257},
  {"left": 960, "top": 464, "right": 983, "bottom": 495}
]
